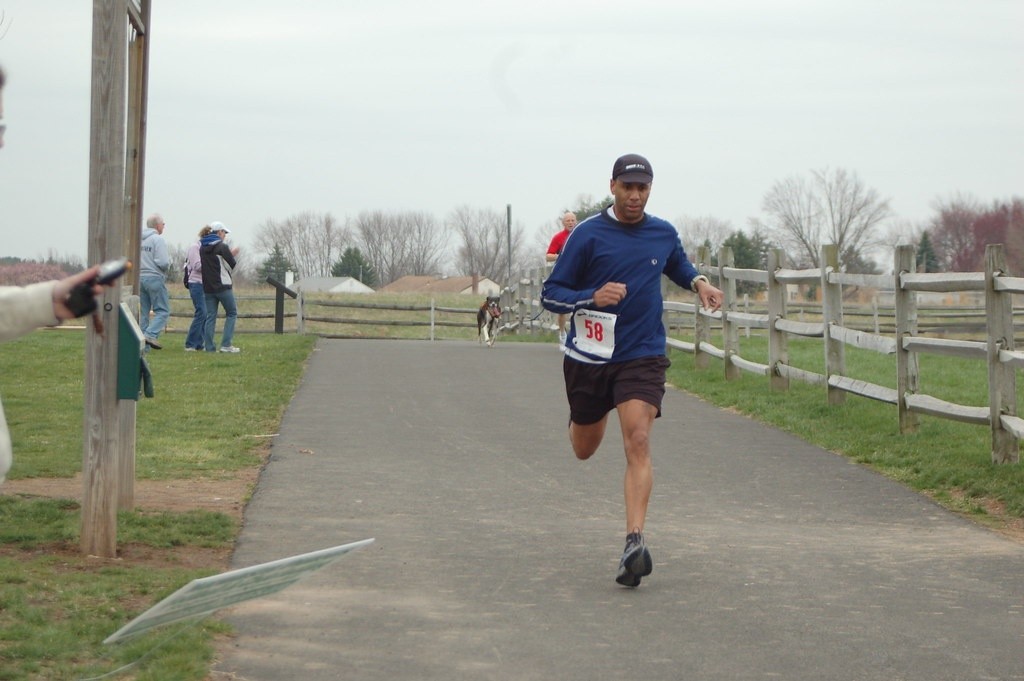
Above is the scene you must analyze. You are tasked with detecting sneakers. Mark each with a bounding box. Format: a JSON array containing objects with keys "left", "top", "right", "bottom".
[{"left": 616, "top": 527, "right": 652, "bottom": 586}]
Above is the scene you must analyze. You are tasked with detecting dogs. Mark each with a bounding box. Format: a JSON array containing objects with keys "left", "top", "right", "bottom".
[{"left": 477, "top": 296, "right": 502, "bottom": 347}]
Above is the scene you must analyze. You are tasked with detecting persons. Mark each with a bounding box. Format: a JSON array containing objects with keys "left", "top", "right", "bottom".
[
  {"left": 545, "top": 211, "right": 578, "bottom": 346},
  {"left": 539, "top": 152, "right": 724, "bottom": 589},
  {"left": 137, "top": 212, "right": 171, "bottom": 353},
  {"left": 199, "top": 221, "right": 240, "bottom": 353},
  {"left": 183, "top": 224, "right": 213, "bottom": 352},
  {"left": 1, "top": 65, "right": 131, "bottom": 506}
]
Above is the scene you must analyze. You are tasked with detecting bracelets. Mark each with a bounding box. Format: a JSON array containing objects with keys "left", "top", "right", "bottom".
[{"left": 690, "top": 274, "right": 710, "bottom": 293}]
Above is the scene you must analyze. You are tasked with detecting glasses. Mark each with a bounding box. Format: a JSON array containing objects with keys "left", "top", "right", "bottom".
[{"left": 162, "top": 223, "right": 165, "bottom": 225}]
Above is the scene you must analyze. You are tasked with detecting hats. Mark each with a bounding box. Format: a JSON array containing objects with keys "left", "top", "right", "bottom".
[
  {"left": 613, "top": 154, "right": 653, "bottom": 183},
  {"left": 210, "top": 221, "right": 230, "bottom": 234}
]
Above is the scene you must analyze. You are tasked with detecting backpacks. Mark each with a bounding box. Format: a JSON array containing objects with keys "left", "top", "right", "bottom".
[{"left": 183, "top": 258, "right": 192, "bottom": 289}]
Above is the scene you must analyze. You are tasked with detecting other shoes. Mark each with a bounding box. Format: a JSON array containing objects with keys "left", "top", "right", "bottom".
[
  {"left": 185, "top": 348, "right": 206, "bottom": 351},
  {"left": 559, "top": 331, "right": 567, "bottom": 352},
  {"left": 220, "top": 346, "right": 240, "bottom": 353},
  {"left": 143, "top": 334, "right": 162, "bottom": 349}
]
